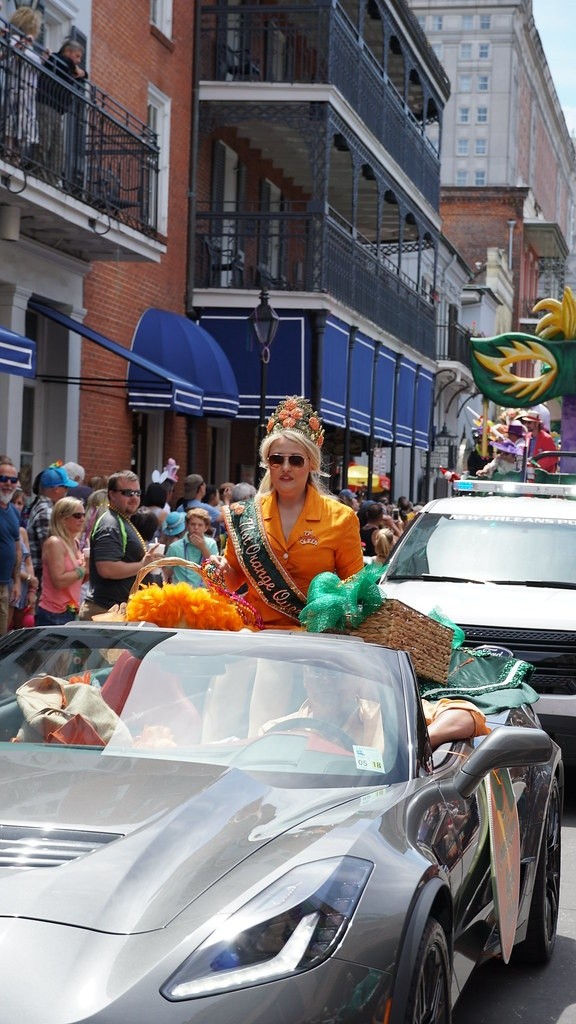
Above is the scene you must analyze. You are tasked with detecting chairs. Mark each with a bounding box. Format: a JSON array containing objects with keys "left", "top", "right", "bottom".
[
  {"left": 102, "top": 650, "right": 202, "bottom": 738},
  {"left": 256, "top": 268, "right": 290, "bottom": 291},
  {"left": 213, "top": 42, "right": 261, "bottom": 83},
  {"left": 87, "top": 165, "right": 143, "bottom": 220},
  {"left": 201, "top": 236, "right": 244, "bottom": 289}
]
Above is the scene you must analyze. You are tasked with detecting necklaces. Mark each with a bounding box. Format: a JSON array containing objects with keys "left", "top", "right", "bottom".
[{"left": 107, "top": 504, "right": 147, "bottom": 552}]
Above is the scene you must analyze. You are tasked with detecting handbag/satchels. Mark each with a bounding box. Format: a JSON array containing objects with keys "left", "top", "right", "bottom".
[{"left": 15, "top": 673, "right": 133, "bottom": 747}]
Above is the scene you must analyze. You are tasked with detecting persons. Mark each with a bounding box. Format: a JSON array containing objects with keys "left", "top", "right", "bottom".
[
  {"left": 466, "top": 404, "right": 558, "bottom": 497},
  {"left": 201, "top": 396, "right": 364, "bottom": 744},
  {"left": 259, "top": 664, "right": 492, "bottom": 753},
  {"left": 33, "top": 40, "right": 89, "bottom": 186},
  {"left": 4, "top": 7, "right": 52, "bottom": 164},
  {"left": 0, "top": 454, "right": 256, "bottom": 638},
  {"left": 340, "top": 489, "right": 423, "bottom": 563}
]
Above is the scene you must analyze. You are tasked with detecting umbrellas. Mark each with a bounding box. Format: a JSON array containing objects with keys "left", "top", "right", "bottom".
[{"left": 347, "top": 462, "right": 390, "bottom": 493}]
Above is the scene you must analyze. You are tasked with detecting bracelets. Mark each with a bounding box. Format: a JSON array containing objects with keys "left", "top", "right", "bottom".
[
  {"left": 75, "top": 568, "right": 84, "bottom": 579},
  {"left": 29, "top": 586, "right": 37, "bottom": 593},
  {"left": 27, "top": 575, "right": 31, "bottom": 581}
]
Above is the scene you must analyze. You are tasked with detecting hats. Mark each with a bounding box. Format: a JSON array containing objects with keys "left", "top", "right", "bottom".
[
  {"left": 505, "top": 425, "right": 525, "bottom": 437},
  {"left": 361, "top": 500, "right": 382, "bottom": 512},
  {"left": 41, "top": 468, "right": 80, "bottom": 487},
  {"left": 162, "top": 511, "right": 187, "bottom": 535},
  {"left": 338, "top": 489, "right": 358, "bottom": 498},
  {"left": 522, "top": 411, "right": 543, "bottom": 422},
  {"left": 489, "top": 439, "right": 518, "bottom": 455},
  {"left": 183, "top": 474, "right": 204, "bottom": 500},
  {"left": 513, "top": 410, "right": 528, "bottom": 420},
  {"left": 62, "top": 462, "right": 86, "bottom": 480}
]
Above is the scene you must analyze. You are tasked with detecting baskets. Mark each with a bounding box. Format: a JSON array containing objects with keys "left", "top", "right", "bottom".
[{"left": 315, "top": 598, "right": 455, "bottom": 685}]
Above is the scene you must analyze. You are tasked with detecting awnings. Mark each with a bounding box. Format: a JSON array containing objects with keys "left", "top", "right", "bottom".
[
  {"left": 0, "top": 326, "right": 37, "bottom": 378},
  {"left": 27, "top": 301, "right": 204, "bottom": 417},
  {"left": 128, "top": 308, "right": 240, "bottom": 418}
]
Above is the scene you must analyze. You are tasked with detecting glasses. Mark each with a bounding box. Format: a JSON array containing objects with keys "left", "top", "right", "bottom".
[
  {"left": 70, "top": 513, "right": 86, "bottom": 519},
  {"left": 111, "top": 489, "right": 142, "bottom": 497},
  {"left": 267, "top": 454, "right": 310, "bottom": 468},
  {"left": 0, "top": 475, "right": 19, "bottom": 484}
]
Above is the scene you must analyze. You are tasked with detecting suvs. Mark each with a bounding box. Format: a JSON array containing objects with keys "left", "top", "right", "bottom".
[{"left": 357, "top": 479, "right": 576, "bottom": 813}]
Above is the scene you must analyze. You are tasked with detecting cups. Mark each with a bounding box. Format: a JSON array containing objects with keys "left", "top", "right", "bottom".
[
  {"left": 83, "top": 547, "right": 90, "bottom": 558},
  {"left": 148, "top": 544, "right": 165, "bottom": 574}
]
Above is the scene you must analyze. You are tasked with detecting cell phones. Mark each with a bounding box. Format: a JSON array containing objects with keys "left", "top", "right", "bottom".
[{"left": 219, "top": 489, "right": 225, "bottom": 493}]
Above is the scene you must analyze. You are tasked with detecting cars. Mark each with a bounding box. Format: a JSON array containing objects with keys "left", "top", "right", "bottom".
[{"left": 0, "top": 621, "right": 564, "bottom": 1024}]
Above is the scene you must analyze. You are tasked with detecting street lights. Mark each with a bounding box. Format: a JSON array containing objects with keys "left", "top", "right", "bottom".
[{"left": 247, "top": 287, "right": 280, "bottom": 491}]
